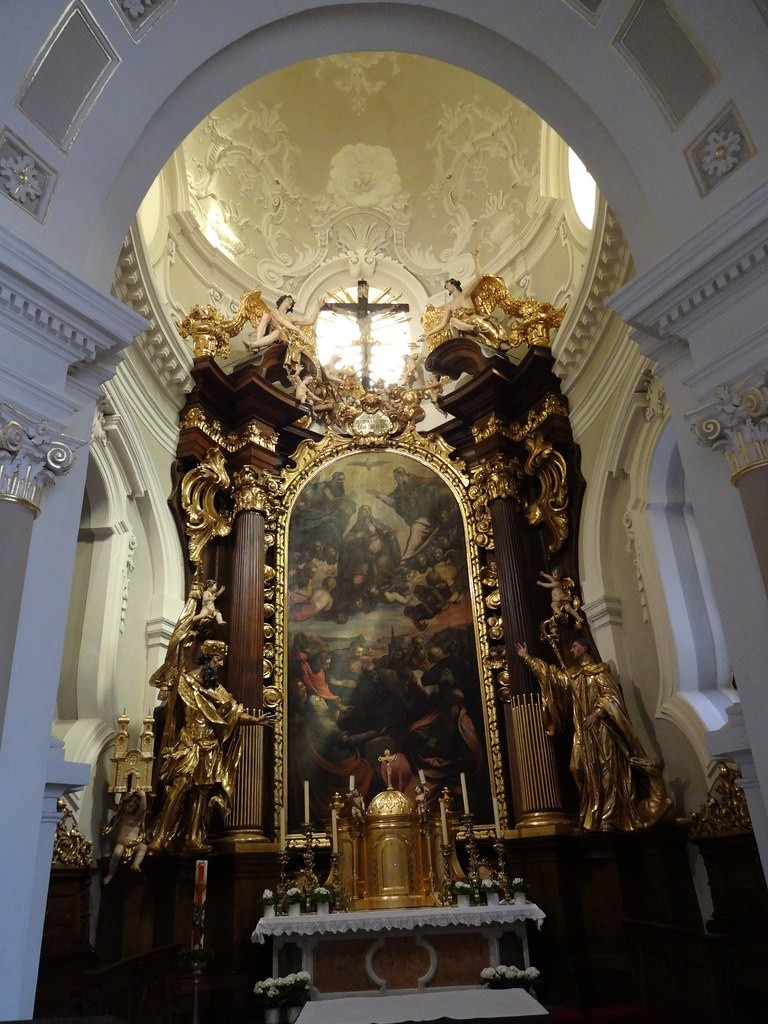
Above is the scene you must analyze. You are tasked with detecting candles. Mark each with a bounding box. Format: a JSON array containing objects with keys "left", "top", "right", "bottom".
[
  {"left": 493, "top": 797, "right": 502, "bottom": 839},
  {"left": 331, "top": 809, "right": 339, "bottom": 853},
  {"left": 304, "top": 780, "right": 310, "bottom": 823},
  {"left": 460, "top": 772, "right": 470, "bottom": 813},
  {"left": 440, "top": 801, "right": 449, "bottom": 845},
  {"left": 279, "top": 806, "right": 286, "bottom": 850}
]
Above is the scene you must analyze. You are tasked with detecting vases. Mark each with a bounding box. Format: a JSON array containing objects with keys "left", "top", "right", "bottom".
[
  {"left": 457, "top": 895, "right": 470, "bottom": 907},
  {"left": 513, "top": 892, "right": 526, "bottom": 905},
  {"left": 287, "top": 1006, "right": 302, "bottom": 1024},
  {"left": 264, "top": 904, "right": 276, "bottom": 918},
  {"left": 317, "top": 902, "right": 329, "bottom": 915},
  {"left": 263, "top": 1007, "right": 281, "bottom": 1024},
  {"left": 487, "top": 894, "right": 499, "bottom": 907},
  {"left": 289, "top": 903, "right": 300, "bottom": 917}
]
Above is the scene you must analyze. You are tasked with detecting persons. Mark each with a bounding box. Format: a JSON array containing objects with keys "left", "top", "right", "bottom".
[
  {"left": 414, "top": 786, "right": 430, "bottom": 823},
  {"left": 148, "top": 640, "right": 278, "bottom": 854},
  {"left": 346, "top": 789, "right": 366, "bottom": 824},
  {"left": 241, "top": 294, "right": 327, "bottom": 353},
  {"left": 102, "top": 787, "right": 148, "bottom": 885},
  {"left": 417, "top": 246, "right": 512, "bottom": 352},
  {"left": 513, "top": 639, "right": 676, "bottom": 835},
  {"left": 294, "top": 364, "right": 323, "bottom": 417},
  {"left": 193, "top": 579, "right": 226, "bottom": 625},
  {"left": 421, "top": 372, "right": 447, "bottom": 419},
  {"left": 537, "top": 566, "right": 584, "bottom": 623},
  {"left": 324, "top": 353, "right": 424, "bottom": 424}
]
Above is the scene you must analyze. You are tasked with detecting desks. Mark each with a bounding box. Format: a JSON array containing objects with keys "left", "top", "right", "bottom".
[{"left": 249, "top": 903, "right": 538, "bottom": 1000}]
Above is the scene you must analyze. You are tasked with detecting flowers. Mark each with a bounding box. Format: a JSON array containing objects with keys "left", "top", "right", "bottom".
[
  {"left": 284, "top": 887, "right": 307, "bottom": 905},
  {"left": 480, "top": 965, "right": 545, "bottom": 990},
  {"left": 285, "top": 971, "right": 312, "bottom": 1007},
  {"left": 454, "top": 881, "right": 475, "bottom": 895},
  {"left": 311, "top": 886, "right": 334, "bottom": 905},
  {"left": 256, "top": 888, "right": 275, "bottom": 905},
  {"left": 481, "top": 878, "right": 503, "bottom": 894},
  {"left": 506, "top": 877, "right": 532, "bottom": 895},
  {"left": 255, "top": 977, "right": 288, "bottom": 1008}
]
